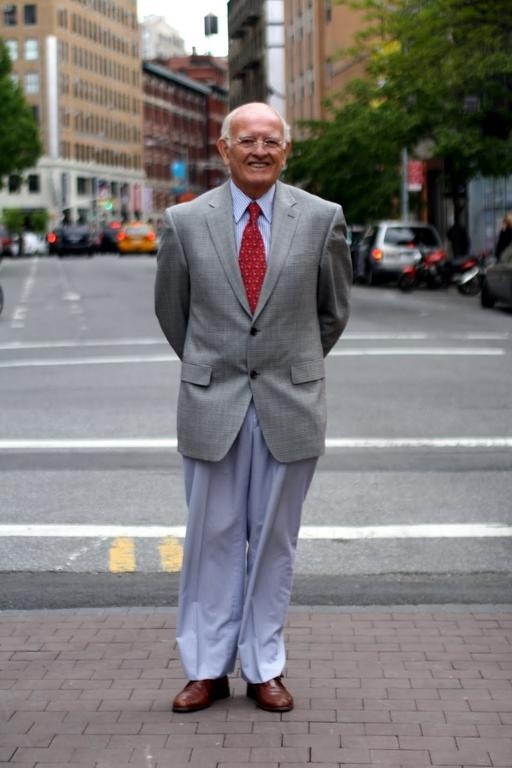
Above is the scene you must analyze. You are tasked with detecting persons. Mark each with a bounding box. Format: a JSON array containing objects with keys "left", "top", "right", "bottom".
[{"left": 153, "top": 100, "right": 355, "bottom": 717}]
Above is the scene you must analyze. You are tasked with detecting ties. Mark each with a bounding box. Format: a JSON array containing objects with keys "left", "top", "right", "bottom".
[{"left": 238, "top": 200, "right": 268, "bottom": 317}]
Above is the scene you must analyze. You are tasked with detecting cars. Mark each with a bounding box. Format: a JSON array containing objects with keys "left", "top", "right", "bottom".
[
  {"left": 479, "top": 244, "right": 512, "bottom": 312},
  {"left": 351, "top": 220, "right": 444, "bottom": 285},
  {"left": 46, "top": 220, "right": 162, "bottom": 258}
]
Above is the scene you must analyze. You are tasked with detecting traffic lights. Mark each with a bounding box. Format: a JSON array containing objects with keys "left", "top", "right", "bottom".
[{"left": 97, "top": 199, "right": 121, "bottom": 210}]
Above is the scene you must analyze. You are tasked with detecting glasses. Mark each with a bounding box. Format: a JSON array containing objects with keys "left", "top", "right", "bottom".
[{"left": 226, "top": 135, "right": 286, "bottom": 149}]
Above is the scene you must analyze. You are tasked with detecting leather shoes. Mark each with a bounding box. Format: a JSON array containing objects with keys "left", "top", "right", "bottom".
[
  {"left": 244, "top": 676, "right": 294, "bottom": 712},
  {"left": 171, "top": 675, "right": 232, "bottom": 714}
]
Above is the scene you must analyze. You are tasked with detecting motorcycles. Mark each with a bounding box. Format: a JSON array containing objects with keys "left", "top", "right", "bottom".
[{"left": 400, "top": 247, "right": 482, "bottom": 297}]
